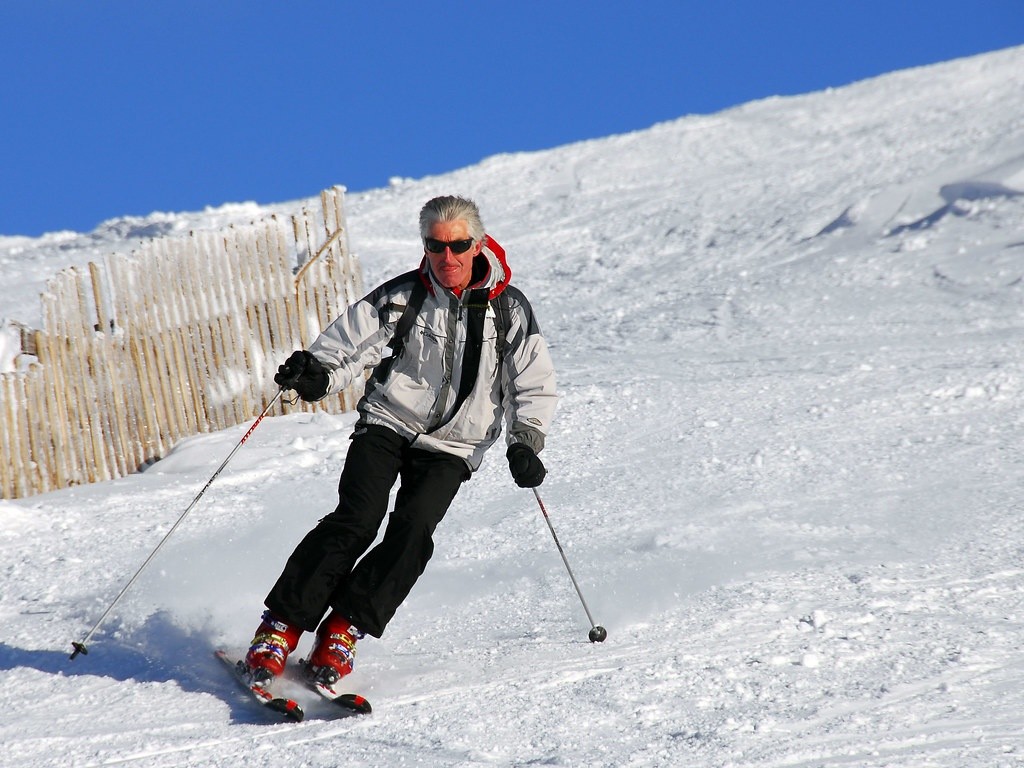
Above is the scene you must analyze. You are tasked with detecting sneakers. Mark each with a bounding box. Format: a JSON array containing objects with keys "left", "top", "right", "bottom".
[
  {"left": 303, "top": 610, "right": 367, "bottom": 684},
  {"left": 240, "top": 610, "right": 306, "bottom": 681}
]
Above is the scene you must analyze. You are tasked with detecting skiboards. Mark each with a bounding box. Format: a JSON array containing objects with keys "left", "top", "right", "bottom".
[
  {"left": 298, "top": 658, "right": 372, "bottom": 714},
  {"left": 213, "top": 644, "right": 304, "bottom": 722}
]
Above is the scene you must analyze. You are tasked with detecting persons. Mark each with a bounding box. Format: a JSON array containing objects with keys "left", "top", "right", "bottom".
[{"left": 236, "top": 195, "right": 557, "bottom": 689}]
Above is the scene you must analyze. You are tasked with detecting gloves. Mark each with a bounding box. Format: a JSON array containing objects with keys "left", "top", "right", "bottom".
[
  {"left": 274, "top": 350, "right": 329, "bottom": 403},
  {"left": 506, "top": 442, "right": 546, "bottom": 488}
]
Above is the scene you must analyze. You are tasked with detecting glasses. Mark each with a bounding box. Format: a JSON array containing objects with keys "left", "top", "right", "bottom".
[{"left": 424, "top": 235, "right": 473, "bottom": 254}]
